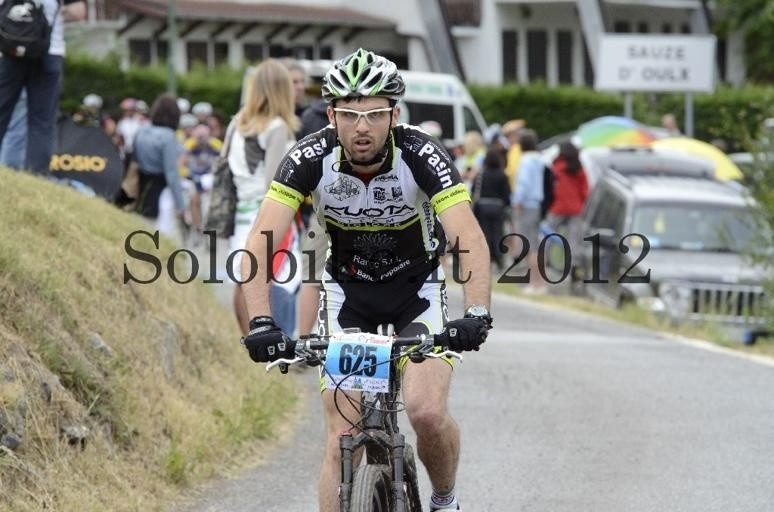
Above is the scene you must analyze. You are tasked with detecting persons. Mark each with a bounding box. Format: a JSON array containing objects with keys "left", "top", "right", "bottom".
[
  {"left": 234, "top": 51, "right": 496, "bottom": 512},
  {"left": 222, "top": 59, "right": 296, "bottom": 338},
  {"left": 71, "top": 86, "right": 743, "bottom": 285},
  {"left": 0, "top": 0, "right": 87, "bottom": 200}
]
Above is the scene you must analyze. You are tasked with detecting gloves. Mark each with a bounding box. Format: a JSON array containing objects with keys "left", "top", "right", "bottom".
[
  {"left": 442, "top": 314, "right": 493, "bottom": 359},
  {"left": 244, "top": 315, "right": 292, "bottom": 374}
]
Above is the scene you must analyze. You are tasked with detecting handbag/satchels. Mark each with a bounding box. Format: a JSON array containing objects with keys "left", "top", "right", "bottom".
[
  {"left": 121, "top": 161, "right": 140, "bottom": 199},
  {"left": 203, "top": 123, "right": 236, "bottom": 238}
]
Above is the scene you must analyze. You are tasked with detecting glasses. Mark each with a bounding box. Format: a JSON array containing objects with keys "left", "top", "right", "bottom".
[{"left": 333, "top": 107, "right": 393, "bottom": 128}]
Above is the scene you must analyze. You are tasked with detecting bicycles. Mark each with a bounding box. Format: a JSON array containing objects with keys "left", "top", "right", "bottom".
[{"left": 231, "top": 318, "right": 495, "bottom": 512}]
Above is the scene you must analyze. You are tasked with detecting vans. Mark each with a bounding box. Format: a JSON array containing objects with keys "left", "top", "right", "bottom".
[{"left": 238, "top": 57, "right": 500, "bottom": 161}]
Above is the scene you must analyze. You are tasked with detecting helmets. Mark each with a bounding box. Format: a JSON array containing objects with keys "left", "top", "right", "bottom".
[
  {"left": 502, "top": 118, "right": 527, "bottom": 135},
  {"left": 320, "top": 47, "right": 406, "bottom": 104},
  {"left": 120, "top": 97, "right": 213, "bottom": 130},
  {"left": 82, "top": 92, "right": 104, "bottom": 108}
]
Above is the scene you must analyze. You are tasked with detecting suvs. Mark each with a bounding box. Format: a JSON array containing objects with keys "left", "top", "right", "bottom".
[{"left": 563, "top": 157, "right": 773, "bottom": 347}]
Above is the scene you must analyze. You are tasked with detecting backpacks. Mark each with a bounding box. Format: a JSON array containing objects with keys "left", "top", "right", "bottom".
[{"left": 0, "top": 0, "right": 60, "bottom": 59}]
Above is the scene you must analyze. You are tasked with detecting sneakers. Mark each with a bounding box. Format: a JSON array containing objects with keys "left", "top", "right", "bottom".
[{"left": 429, "top": 499, "right": 461, "bottom": 512}]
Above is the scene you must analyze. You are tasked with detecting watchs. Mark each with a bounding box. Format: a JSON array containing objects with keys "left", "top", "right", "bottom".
[{"left": 463, "top": 304, "right": 491, "bottom": 324}]
